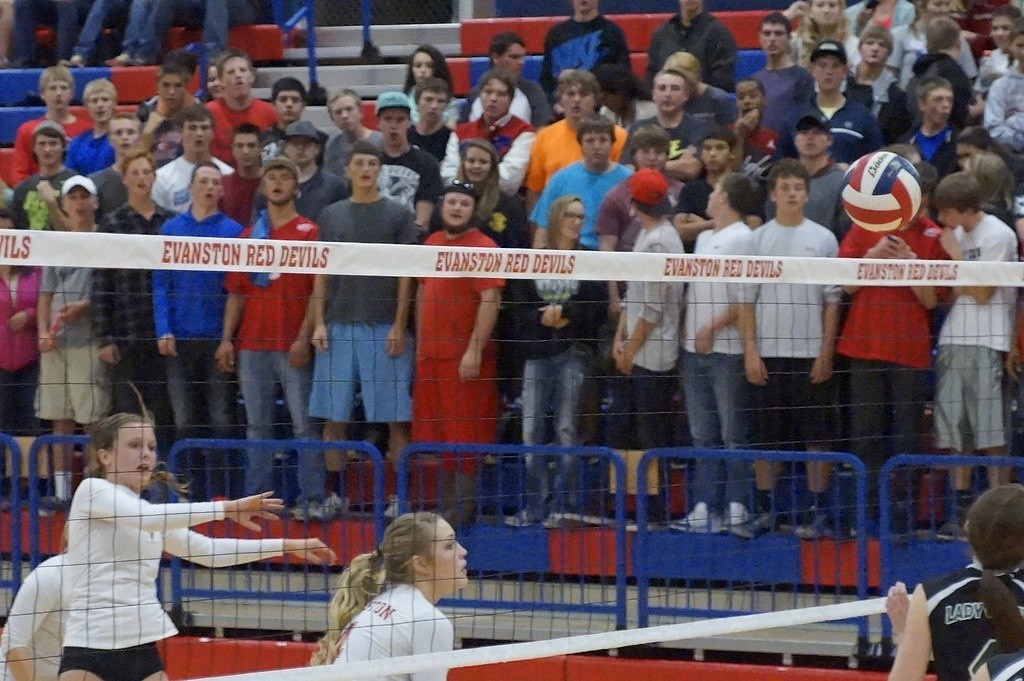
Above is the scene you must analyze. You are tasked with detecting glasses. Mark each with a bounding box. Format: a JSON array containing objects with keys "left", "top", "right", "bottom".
[{"left": 563, "top": 209, "right": 586, "bottom": 220}]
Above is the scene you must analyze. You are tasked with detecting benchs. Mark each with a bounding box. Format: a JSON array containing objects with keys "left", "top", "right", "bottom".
[{"left": 0, "top": 7, "right": 1005, "bottom": 588}]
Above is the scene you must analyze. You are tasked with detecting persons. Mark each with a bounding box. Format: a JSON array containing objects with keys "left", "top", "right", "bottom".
[
  {"left": 886, "top": 484, "right": 1024, "bottom": 681},
  {"left": 0, "top": 547, "right": 76, "bottom": 681},
  {"left": 56, "top": 412, "right": 338, "bottom": 681},
  {"left": 0, "top": 0, "right": 1024, "bottom": 539},
  {"left": 310, "top": 511, "right": 468, "bottom": 681}
]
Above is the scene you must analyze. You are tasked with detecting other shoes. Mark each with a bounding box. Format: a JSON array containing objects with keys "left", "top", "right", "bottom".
[
  {"left": 0, "top": 53, "right": 42, "bottom": 68},
  {"left": 102, "top": 51, "right": 129, "bottom": 67},
  {"left": 37, "top": 494, "right": 73, "bottom": 513},
  {"left": 57, "top": 52, "right": 91, "bottom": 68},
  {"left": 207, "top": 45, "right": 222, "bottom": 65},
  {"left": 429, "top": 502, "right": 478, "bottom": 530},
  {"left": 124, "top": 49, "right": 159, "bottom": 66}
]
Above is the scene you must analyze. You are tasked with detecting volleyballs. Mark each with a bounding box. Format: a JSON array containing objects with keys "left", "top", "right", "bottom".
[{"left": 839, "top": 150, "right": 924, "bottom": 233}]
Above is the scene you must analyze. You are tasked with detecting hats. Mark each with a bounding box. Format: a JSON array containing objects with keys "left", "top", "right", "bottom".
[
  {"left": 283, "top": 119, "right": 323, "bottom": 143},
  {"left": 62, "top": 176, "right": 98, "bottom": 198},
  {"left": 435, "top": 178, "right": 478, "bottom": 205},
  {"left": 631, "top": 167, "right": 669, "bottom": 205},
  {"left": 809, "top": 39, "right": 847, "bottom": 65},
  {"left": 34, "top": 119, "right": 66, "bottom": 141},
  {"left": 795, "top": 110, "right": 832, "bottom": 133},
  {"left": 256, "top": 159, "right": 298, "bottom": 185},
  {"left": 374, "top": 92, "right": 413, "bottom": 120}
]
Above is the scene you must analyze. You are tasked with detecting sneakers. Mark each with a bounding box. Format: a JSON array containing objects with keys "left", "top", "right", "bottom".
[
  {"left": 935, "top": 507, "right": 967, "bottom": 540},
  {"left": 288, "top": 497, "right": 321, "bottom": 520},
  {"left": 730, "top": 507, "right": 774, "bottom": 538},
  {"left": 779, "top": 504, "right": 837, "bottom": 537},
  {"left": 541, "top": 499, "right": 599, "bottom": 528},
  {"left": 314, "top": 494, "right": 349, "bottom": 522},
  {"left": 676, "top": 499, "right": 724, "bottom": 529},
  {"left": 501, "top": 504, "right": 551, "bottom": 524},
  {"left": 722, "top": 501, "right": 750, "bottom": 527},
  {"left": 383, "top": 494, "right": 413, "bottom": 518}
]
